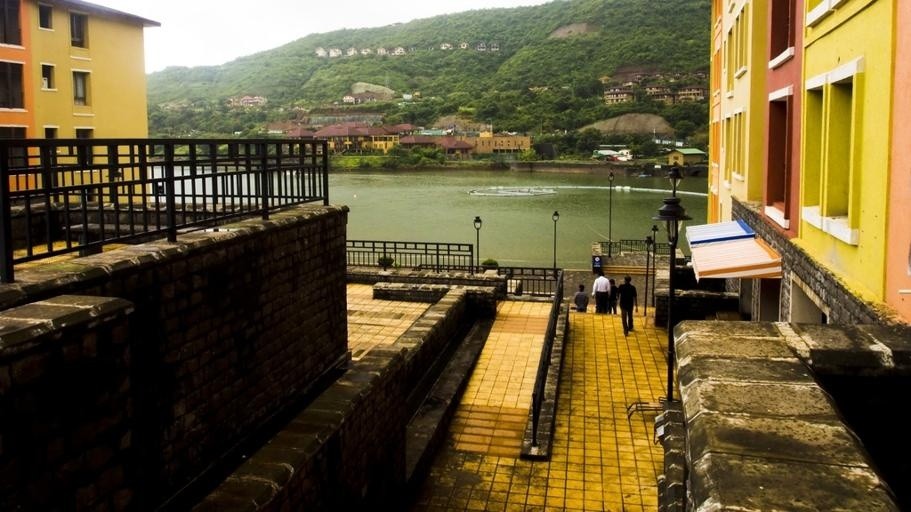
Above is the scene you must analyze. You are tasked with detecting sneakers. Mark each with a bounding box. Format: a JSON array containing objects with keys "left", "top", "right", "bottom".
[{"left": 625, "top": 326, "right": 633, "bottom": 335}]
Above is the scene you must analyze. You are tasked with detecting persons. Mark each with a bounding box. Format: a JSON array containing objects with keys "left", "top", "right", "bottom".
[
  {"left": 592, "top": 271, "right": 611, "bottom": 314},
  {"left": 574, "top": 285, "right": 588, "bottom": 311},
  {"left": 618, "top": 276, "right": 638, "bottom": 336},
  {"left": 608, "top": 279, "right": 619, "bottom": 314}
]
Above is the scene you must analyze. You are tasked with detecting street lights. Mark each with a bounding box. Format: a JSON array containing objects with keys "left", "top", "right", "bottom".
[
  {"left": 643, "top": 162, "right": 693, "bottom": 403},
  {"left": 551, "top": 210, "right": 560, "bottom": 279},
  {"left": 474, "top": 215, "right": 482, "bottom": 269},
  {"left": 607, "top": 166, "right": 615, "bottom": 258}
]
